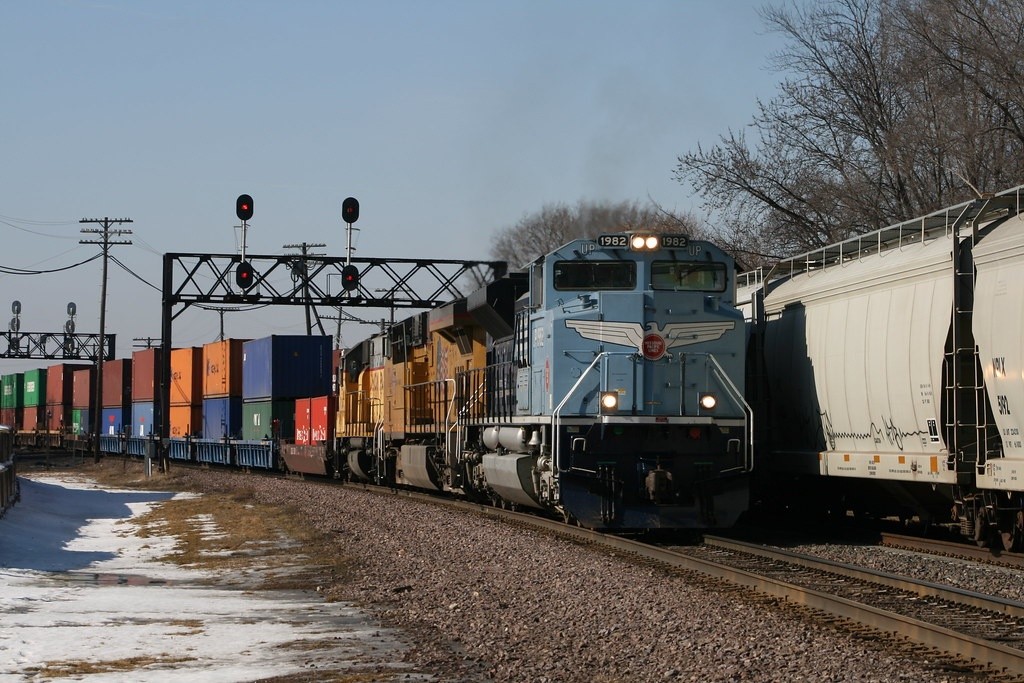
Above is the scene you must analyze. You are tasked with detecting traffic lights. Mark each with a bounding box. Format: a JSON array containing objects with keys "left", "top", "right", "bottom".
[
  {"left": 236, "top": 262, "right": 253, "bottom": 288},
  {"left": 341, "top": 264, "right": 358, "bottom": 291},
  {"left": 236, "top": 194, "right": 254, "bottom": 220},
  {"left": 342, "top": 197, "right": 359, "bottom": 223}
]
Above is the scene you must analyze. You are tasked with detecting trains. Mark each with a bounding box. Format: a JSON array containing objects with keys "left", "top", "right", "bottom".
[
  {"left": 0, "top": 229, "right": 757, "bottom": 534},
  {"left": 733, "top": 183, "right": 1024, "bottom": 556}
]
